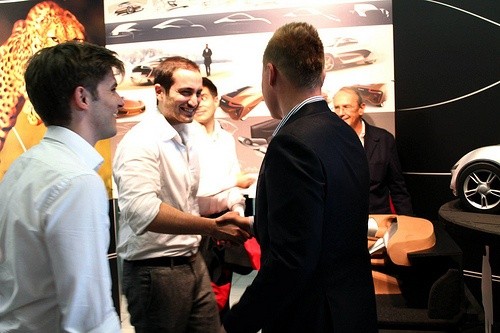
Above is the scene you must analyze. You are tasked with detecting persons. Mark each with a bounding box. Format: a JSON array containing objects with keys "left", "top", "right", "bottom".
[
  {"left": 215, "top": 19, "right": 380, "bottom": 333},
  {"left": 332, "top": 88, "right": 414, "bottom": 217},
  {"left": 0, "top": 39, "right": 126, "bottom": 333},
  {"left": 193, "top": 77, "right": 255, "bottom": 320},
  {"left": 202, "top": 42, "right": 213, "bottom": 76},
  {"left": 111, "top": 54, "right": 250, "bottom": 333}
]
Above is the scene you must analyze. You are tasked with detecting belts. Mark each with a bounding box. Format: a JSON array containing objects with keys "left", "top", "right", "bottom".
[{"left": 124, "top": 247, "right": 199, "bottom": 267}]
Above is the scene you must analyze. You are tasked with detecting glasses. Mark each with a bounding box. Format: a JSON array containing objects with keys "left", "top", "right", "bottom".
[{"left": 333, "top": 103, "right": 355, "bottom": 111}]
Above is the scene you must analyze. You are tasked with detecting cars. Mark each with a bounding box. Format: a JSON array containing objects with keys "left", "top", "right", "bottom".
[
  {"left": 449, "top": 142, "right": 500, "bottom": 214},
  {"left": 115, "top": 1, "right": 142, "bottom": 17}
]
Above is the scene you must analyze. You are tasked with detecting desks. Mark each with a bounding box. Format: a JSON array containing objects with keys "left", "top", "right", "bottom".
[{"left": 440, "top": 199, "right": 500, "bottom": 333}]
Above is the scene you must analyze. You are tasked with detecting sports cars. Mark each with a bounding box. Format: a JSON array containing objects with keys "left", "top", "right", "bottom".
[
  {"left": 323, "top": 35, "right": 376, "bottom": 72},
  {"left": 218, "top": 85, "right": 265, "bottom": 121},
  {"left": 115, "top": 96, "right": 146, "bottom": 119},
  {"left": 350, "top": 82, "right": 391, "bottom": 107},
  {"left": 130, "top": 55, "right": 171, "bottom": 85}
]
